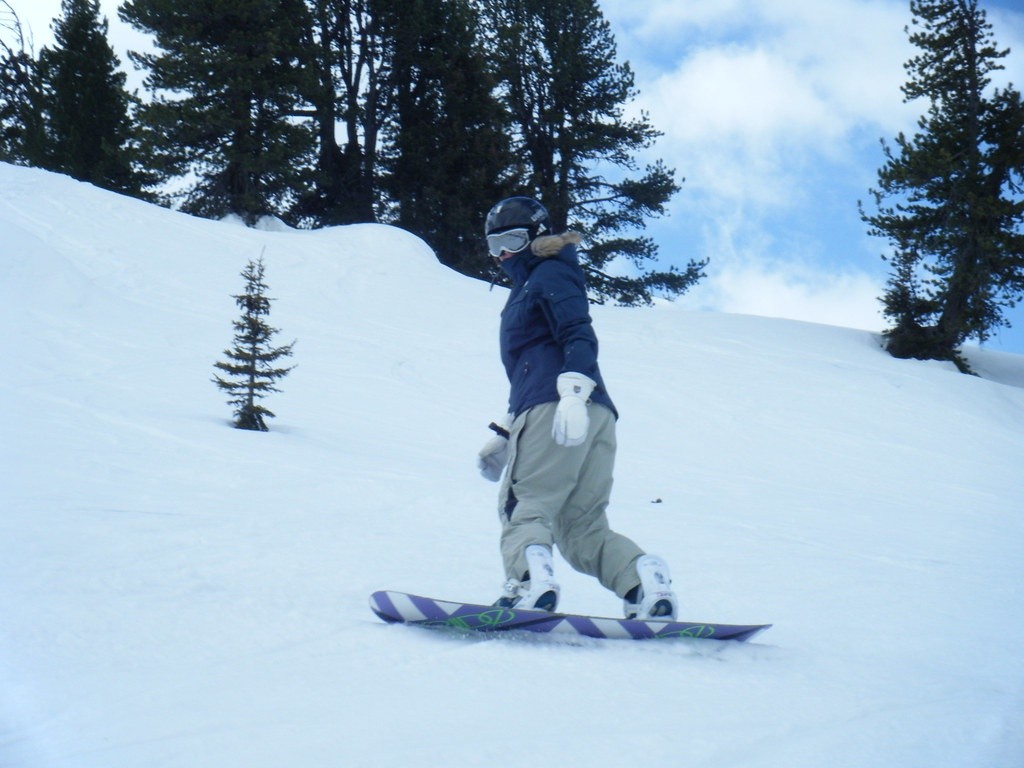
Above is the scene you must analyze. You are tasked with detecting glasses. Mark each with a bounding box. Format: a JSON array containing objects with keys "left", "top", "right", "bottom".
[{"left": 485, "top": 228, "right": 532, "bottom": 257}]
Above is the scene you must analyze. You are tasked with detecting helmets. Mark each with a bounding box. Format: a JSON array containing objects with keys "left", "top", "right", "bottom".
[{"left": 485, "top": 196, "right": 552, "bottom": 241}]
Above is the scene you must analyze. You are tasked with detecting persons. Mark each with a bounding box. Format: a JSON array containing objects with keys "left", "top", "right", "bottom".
[{"left": 477, "top": 197, "right": 679, "bottom": 619}]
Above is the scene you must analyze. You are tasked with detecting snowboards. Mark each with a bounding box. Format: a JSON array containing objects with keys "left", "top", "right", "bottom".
[{"left": 367, "top": 588, "right": 771, "bottom": 641}]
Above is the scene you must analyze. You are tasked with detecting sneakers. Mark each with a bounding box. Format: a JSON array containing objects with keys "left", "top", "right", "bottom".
[
  {"left": 624, "top": 584, "right": 672, "bottom": 620},
  {"left": 491, "top": 570, "right": 555, "bottom": 610}
]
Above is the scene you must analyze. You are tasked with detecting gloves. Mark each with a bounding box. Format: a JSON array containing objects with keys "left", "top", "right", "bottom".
[
  {"left": 552, "top": 372, "right": 597, "bottom": 447},
  {"left": 477, "top": 413, "right": 513, "bottom": 482}
]
